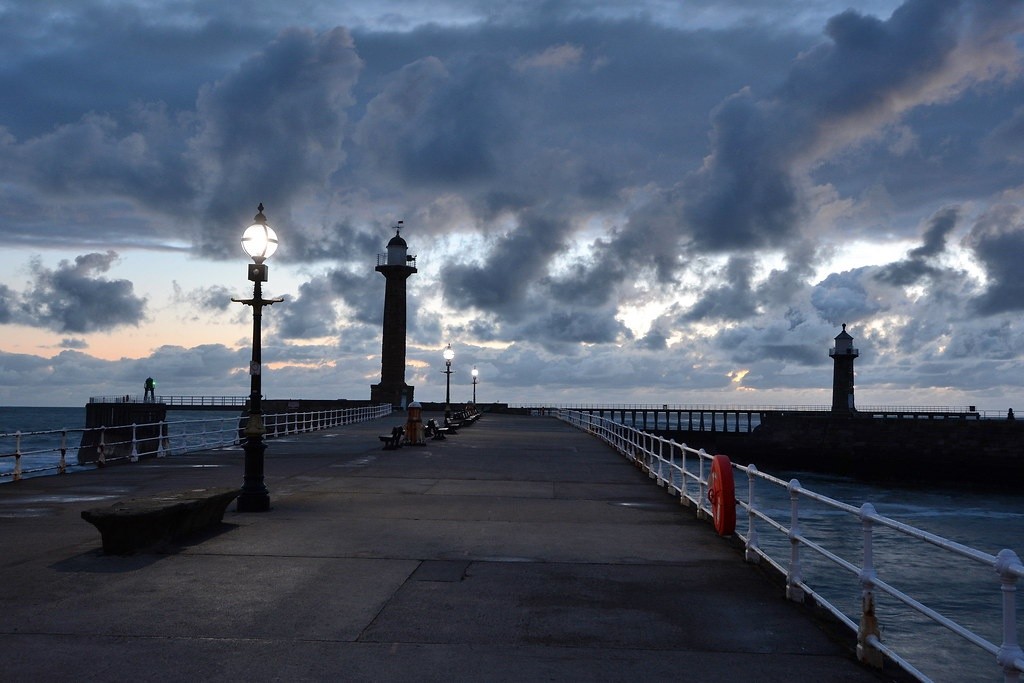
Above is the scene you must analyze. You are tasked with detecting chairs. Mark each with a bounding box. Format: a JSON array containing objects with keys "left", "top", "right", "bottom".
[{"left": 378, "top": 404, "right": 481, "bottom": 451}]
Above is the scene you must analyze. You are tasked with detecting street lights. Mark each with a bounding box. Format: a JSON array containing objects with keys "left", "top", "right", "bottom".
[
  {"left": 231, "top": 201, "right": 286, "bottom": 496},
  {"left": 443, "top": 342, "right": 456, "bottom": 413},
  {"left": 469, "top": 365, "right": 480, "bottom": 408}
]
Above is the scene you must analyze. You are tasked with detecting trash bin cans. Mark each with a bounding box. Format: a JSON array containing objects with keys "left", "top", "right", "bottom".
[{"left": 406, "top": 401, "right": 422, "bottom": 445}]
